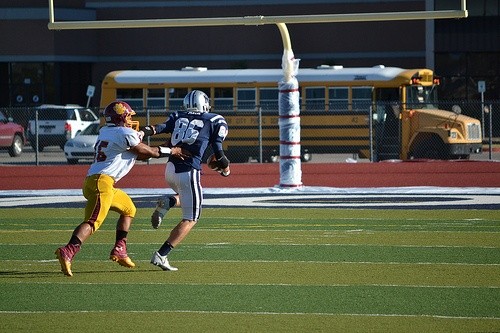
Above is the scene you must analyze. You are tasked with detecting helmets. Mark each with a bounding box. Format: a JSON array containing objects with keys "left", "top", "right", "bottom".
[
  {"left": 183, "top": 90, "right": 210, "bottom": 113},
  {"left": 104, "top": 102, "right": 136, "bottom": 128}
]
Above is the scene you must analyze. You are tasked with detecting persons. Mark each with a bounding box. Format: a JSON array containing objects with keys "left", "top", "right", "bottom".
[
  {"left": 138, "top": 91, "right": 230, "bottom": 270},
  {"left": 55, "top": 101, "right": 182, "bottom": 276}
]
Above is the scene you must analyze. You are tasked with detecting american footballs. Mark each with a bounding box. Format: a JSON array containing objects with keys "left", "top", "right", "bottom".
[{"left": 207, "top": 154, "right": 223, "bottom": 174}]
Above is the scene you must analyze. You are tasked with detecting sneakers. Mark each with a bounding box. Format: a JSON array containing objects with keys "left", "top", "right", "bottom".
[
  {"left": 55, "top": 247, "right": 72, "bottom": 276},
  {"left": 150, "top": 252, "right": 178, "bottom": 272},
  {"left": 110, "top": 248, "right": 135, "bottom": 268},
  {"left": 152, "top": 197, "right": 170, "bottom": 229}
]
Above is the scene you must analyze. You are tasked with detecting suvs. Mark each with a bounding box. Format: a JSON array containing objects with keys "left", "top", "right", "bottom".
[{"left": 26, "top": 104, "right": 99, "bottom": 152}]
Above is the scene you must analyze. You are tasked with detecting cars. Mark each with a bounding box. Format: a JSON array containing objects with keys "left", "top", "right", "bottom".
[
  {"left": 65, "top": 120, "right": 100, "bottom": 164},
  {"left": 0, "top": 111, "right": 26, "bottom": 157}
]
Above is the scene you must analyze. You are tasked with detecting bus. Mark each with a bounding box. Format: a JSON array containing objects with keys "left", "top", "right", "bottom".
[{"left": 101, "top": 65, "right": 482, "bottom": 162}]
